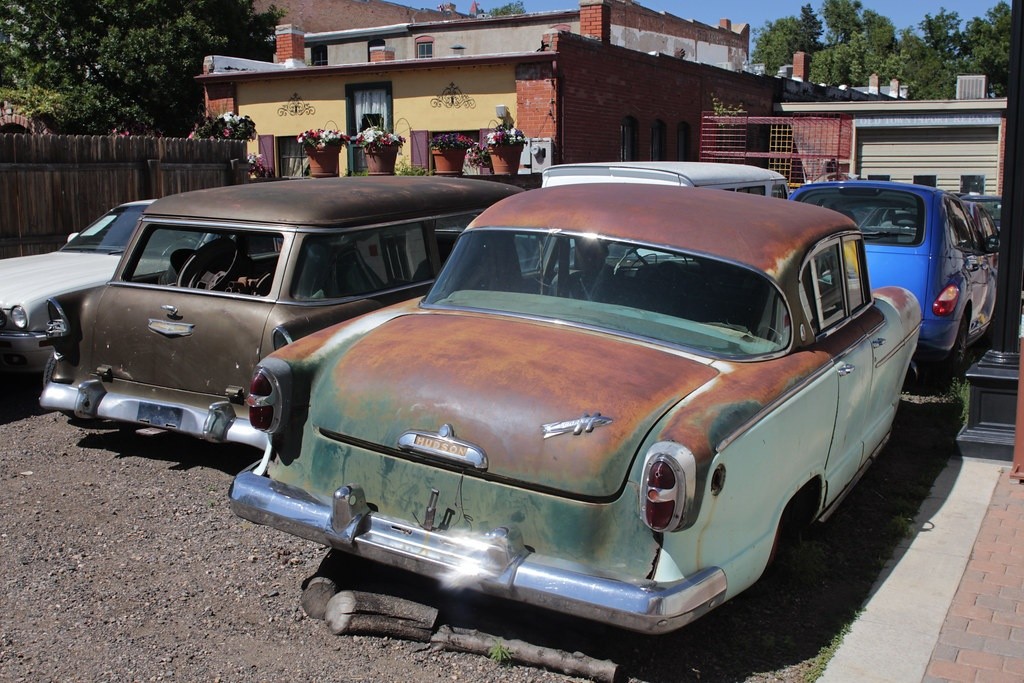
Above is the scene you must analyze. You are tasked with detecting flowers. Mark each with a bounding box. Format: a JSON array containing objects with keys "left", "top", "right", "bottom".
[
  {"left": 486, "top": 110, "right": 526, "bottom": 144},
  {"left": 428, "top": 133, "right": 475, "bottom": 151},
  {"left": 296, "top": 128, "right": 351, "bottom": 149},
  {"left": 355, "top": 126, "right": 406, "bottom": 154},
  {"left": 248, "top": 152, "right": 265, "bottom": 179},
  {"left": 188, "top": 112, "right": 258, "bottom": 141}
]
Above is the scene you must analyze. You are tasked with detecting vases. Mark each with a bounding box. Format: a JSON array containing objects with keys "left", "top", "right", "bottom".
[
  {"left": 303, "top": 145, "right": 342, "bottom": 173},
  {"left": 363, "top": 145, "right": 399, "bottom": 172},
  {"left": 488, "top": 145, "right": 525, "bottom": 175},
  {"left": 432, "top": 148, "right": 467, "bottom": 171}
]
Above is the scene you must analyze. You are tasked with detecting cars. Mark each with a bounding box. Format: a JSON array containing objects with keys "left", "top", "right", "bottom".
[
  {"left": 226, "top": 182, "right": 922, "bottom": 636},
  {"left": 957, "top": 194, "right": 1002, "bottom": 268},
  {"left": 0, "top": 199, "right": 224, "bottom": 376},
  {"left": 540, "top": 161, "right": 791, "bottom": 206},
  {"left": 38, "top": 174, "right": 526, "bottom": 451},
  {"left": 788, "top": 179, "right": 1002, "bottom": 393}
]
